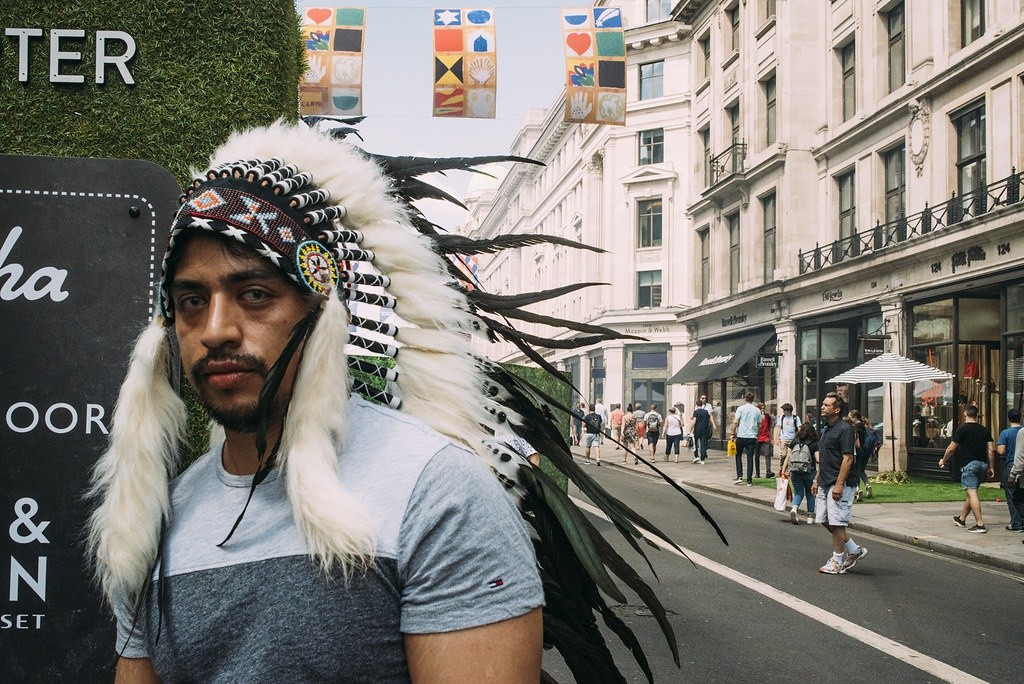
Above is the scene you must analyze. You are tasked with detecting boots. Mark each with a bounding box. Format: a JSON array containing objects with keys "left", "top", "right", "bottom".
[
  {"left": 664, "top": 455, "right": 669, "bottom": 461},
  {"left": 675, "top": 454, "right": 680, "bottom": 463}
]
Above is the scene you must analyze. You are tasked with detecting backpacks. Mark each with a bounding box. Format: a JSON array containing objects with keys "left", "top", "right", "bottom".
[
  {"left": 788, "top": 437, "right": 815, "bottom": 473},
  {"left": 647, "top": 414, "right": 659, "bottom": 430},
  {"left": 588, "top": 414, "right": 601, "bottom": 432}
]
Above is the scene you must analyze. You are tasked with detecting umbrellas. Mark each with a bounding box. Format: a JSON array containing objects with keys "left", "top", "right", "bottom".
[{"left": 825, "top": 347, "right": 953, "bottom": 470}]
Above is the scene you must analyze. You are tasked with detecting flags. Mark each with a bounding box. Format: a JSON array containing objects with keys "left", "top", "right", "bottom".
[
  {"left": 433, "top": 9, "right": 496, "bottom": 118},
  {"left": 297, "top": 6, "right": 366, "bottom": 115},
  {"left": 561, "top": 6, "right": 626, "bottom": 125}
]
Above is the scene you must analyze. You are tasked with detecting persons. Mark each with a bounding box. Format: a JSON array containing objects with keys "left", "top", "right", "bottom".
[
  {"left": 572, "top": 394, "right": 716, "bottom": 467},
  {"left": 730, "top": 392, "right": 875, "bottom": 574},
  {"left": 713, "top": 401, "right": 721, "bottom": 437},
  {"left": 115, "top": 175, "right": 546, "bottom": 684},
  {"left": 913, "top": 406, "right": 926, "bottom": 438},
  {"left": 939, "top": 405, "right": 1024, "bottom": 533}
]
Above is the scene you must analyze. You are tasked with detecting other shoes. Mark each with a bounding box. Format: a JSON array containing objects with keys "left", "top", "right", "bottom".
[
  {"left": 584, "top": 461, "right": 591, "bottom": 465},
  {"left": 650, "top": 458, "right": 655, "bottom": 462},
  {"left": 734, "top": 477, "right": 743, "bottom": 484},
  {"left": 755, "top": 473, "right": 760, "bottom": 478},
  {"left": 694, "top": 457, "right": 699, "bottom": 463},
  {"left": 596, "top": 458, "right": 600, "bottom": 466},
  {"left": 766, "top": 471, "right": 774, "bottom": 478},
  {"left": 747, "top": 480, "right": 753, "bottom": 486},
  {"left": 866, "top": 485, "right": 872, "bottom": 498},
  {"left": 857, "top": 490, "right": 863, "bottom": 502},
  {"left": 700, "top": 460, "right": 705, "bottom": 464}
]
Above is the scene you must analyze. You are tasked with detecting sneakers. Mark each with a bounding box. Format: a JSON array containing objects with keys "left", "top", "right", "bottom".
[
  {"left": 842, "top": 545, "right": 868, "bottom": 568},
  {"left": 819, "top": 558, "right": 846, "bottom": 574},
  {"left": 968, "top": 524, "right": 987, "bottom": 533},
  {"left": 791, "top": 509, "right": 800, "bottom": 525},
  {"left": 1005, "top": 523, "right": 1023, "bottom": 532},
  {"left": 953, "top": 516, "right": 966, "bottom": 527},
  {"left": 807, "top": 517, "right": 814, "bottom": 524}
]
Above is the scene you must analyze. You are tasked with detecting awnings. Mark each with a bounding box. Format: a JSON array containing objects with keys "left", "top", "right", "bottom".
[{"left": 666, "top": 328, "right": 776, "bottom": 384}]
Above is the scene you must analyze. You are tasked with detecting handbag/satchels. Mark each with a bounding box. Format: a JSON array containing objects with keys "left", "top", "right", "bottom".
[
  {"left": 687, "top": 434, "right": 696, "bottom": 452},
  {"left": 862, "top": 428, "right": 875, "bottom": 454},
  {"left": 773, "top": 474, "right": 793, "bottom": 511},
  {"left": 727, "top": 439, "right": 737, "bottom": 457}
]
